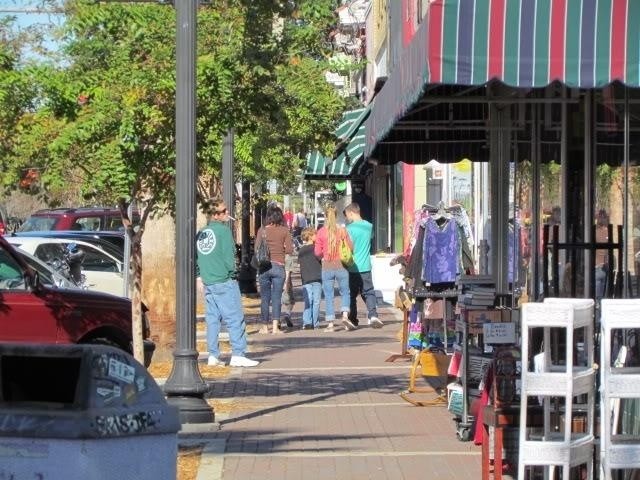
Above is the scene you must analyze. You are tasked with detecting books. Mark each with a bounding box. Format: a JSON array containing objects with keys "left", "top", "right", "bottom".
[
  {"left": 447, "top": 286, "right": 523, "bottom": 423},
  {"left": 557, "top": 414, "right": 602, "bottom": 479}
]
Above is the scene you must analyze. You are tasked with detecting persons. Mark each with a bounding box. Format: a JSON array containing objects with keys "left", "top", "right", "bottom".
[
  {"left": 543, "top": 206, "right": 563, "bottom": 244},
  {"left": 195, "top": 198, "right": 260, "bottom": 367},
  {"left": 595, "top": 210, "right": 611, "bottom": 298},
  {"left": 252, "top": 199, "right": 385, "bottom": 334}
]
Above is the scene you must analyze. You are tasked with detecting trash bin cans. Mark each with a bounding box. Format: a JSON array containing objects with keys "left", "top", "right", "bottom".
[{"left": 0, "top": 340, "right": 182, "bottom": 480}]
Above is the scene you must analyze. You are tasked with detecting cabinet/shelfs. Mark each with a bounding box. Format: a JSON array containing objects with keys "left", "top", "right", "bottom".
[
  {"left": 517, "top": 295, "right": 596, "bottom": 480},
  {"left": 387, "top": 282, "right": 543, "bottom": 480},
  {"left": 599, "top": 298, "right": 640, "bottom": 480},
  {"left": 543, "top": 222, "right": 630, "bottom": 480}
]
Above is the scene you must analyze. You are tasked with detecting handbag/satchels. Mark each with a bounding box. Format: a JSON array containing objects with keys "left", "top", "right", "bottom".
[
  {"left": 251, "top": 240, "right": 272, "bottom": 271},
  {"left": 339, "top": 238, "right": 353, "bottom": 267},
  {"left": 294, "top": 220, "right": 299, "bottom": 229}
]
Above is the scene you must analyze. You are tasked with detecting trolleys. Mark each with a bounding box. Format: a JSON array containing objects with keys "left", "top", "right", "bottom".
[{"left": 451, "top": 317, "right": 478, "bottom": 442}]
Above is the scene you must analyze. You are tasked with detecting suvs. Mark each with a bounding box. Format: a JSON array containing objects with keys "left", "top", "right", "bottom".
[{"left": 0, "top": 235, "right": 156, "bottom": 372}]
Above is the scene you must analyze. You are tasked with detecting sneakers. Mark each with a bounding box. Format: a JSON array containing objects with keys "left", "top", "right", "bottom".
[
  {"left": 259, "top": 313, "right": 384, "bottom": 335},
  {"left": 230, "top": 356, "right": 259, "bottom": 367},
  {"left": 208, "top": 356, "right": 225, "bottom": 367}
]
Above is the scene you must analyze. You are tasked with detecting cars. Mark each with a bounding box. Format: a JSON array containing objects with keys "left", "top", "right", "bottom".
[
  {"left": 317, "top": 210, "right": 325, "bottom": 225},
  {"left": 0, "top": 207, "right": 148, "bottom": 307}
]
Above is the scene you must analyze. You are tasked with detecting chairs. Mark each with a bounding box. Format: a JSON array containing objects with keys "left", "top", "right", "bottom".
[{"left": 70, "top": 223, "right": 85, "bottom": 230}]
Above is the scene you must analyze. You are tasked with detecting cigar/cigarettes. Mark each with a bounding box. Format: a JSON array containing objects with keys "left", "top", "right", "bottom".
[{"left": 227, "top": 215, "right": 235, "bottom": 220}]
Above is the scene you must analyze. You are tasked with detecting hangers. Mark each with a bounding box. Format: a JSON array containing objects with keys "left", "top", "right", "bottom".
[{"left": 424, "top": 199, "right": 455, "bottom": 230}]
